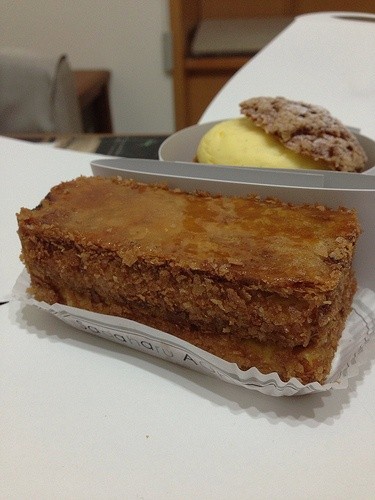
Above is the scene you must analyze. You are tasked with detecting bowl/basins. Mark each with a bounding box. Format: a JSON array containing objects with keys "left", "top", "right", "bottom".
[{"left": 158, "top": 118, "right": 375, "bottom": 174}]
